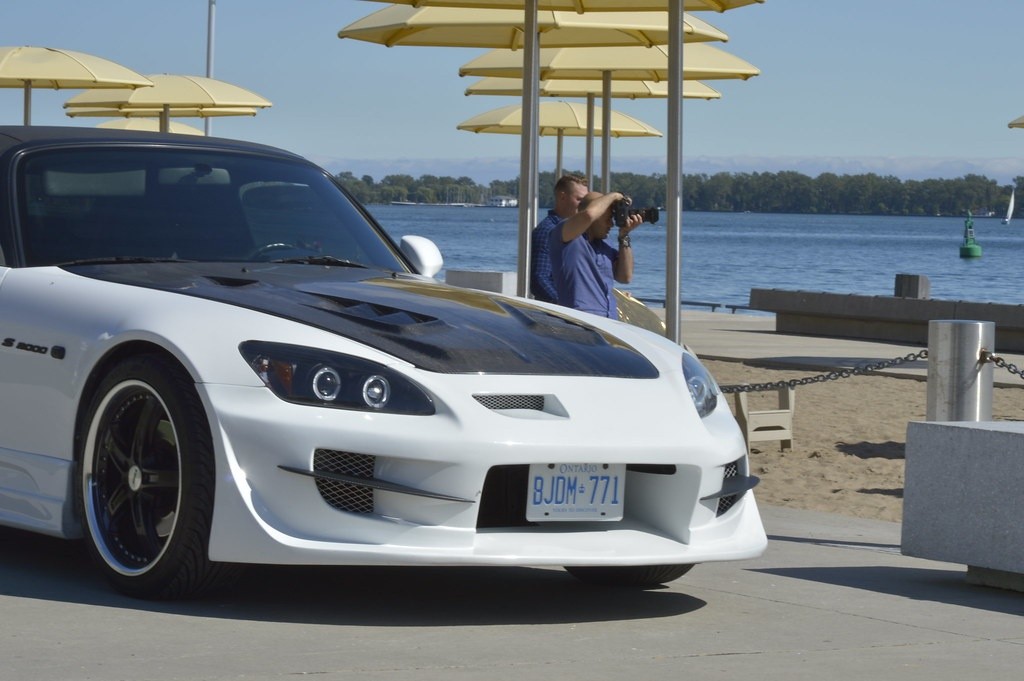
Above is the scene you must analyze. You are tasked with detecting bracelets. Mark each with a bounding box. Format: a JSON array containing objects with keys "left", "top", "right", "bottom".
[{"left": 619, "top": 236, "right": 632, "bottom": 247}]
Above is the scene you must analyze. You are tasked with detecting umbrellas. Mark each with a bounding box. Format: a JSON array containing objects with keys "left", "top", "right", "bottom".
[
  {"left": 337, "top": 0, "right": 766, "bottom": 194},
  {"left": 0, "top": 48, "right": 274, "bottom": 138}
]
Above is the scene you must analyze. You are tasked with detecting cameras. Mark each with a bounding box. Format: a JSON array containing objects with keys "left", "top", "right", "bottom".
[{"left": 613, "top": 200, "right": 659, "bottom": 228}]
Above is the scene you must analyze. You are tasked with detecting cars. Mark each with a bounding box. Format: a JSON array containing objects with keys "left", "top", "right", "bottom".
[{"left": 1, "top": 124, "right": 771, "bottom": 602}]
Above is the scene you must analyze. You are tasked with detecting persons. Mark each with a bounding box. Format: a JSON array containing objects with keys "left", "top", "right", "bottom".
[
  {"left": 530, "top": 174, "right": 588, "bottom": 304},
  {"left": 549, "top": 192, "right": 644, "bottom": 320}
]
